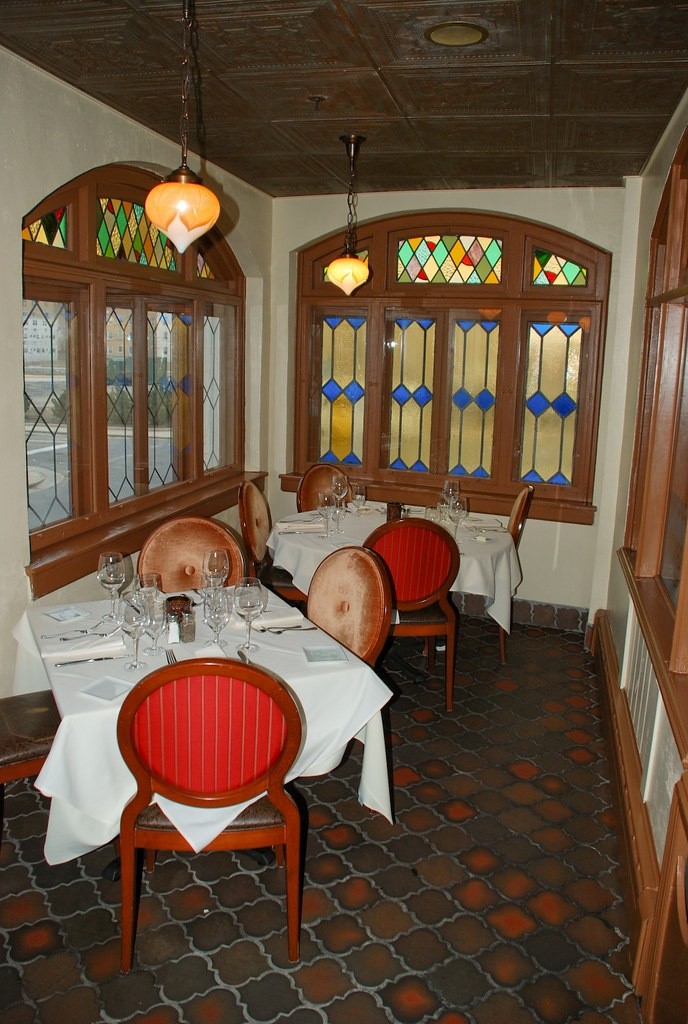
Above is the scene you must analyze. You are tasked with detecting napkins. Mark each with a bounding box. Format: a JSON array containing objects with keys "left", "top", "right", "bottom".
[
  {"left": 265, "top": 501, "right": 522, "bottom": 654},
  {"left": 348, "top": 503, "right": 372, "bottom": 513},
  {"left": 40, "top": 634, "right": 126, "bottom": 657},
  {"left": 464, "top": 519, "right": 501, "bottom": 527},
  {"left": 193, "top": 645, "right": 227, "bottom": 658},
  {"left": 224, "top": 607, "right": 304, "bottom": 627},
  {"left": 274, "top": 521, "right": 324, "bottom": 531}
]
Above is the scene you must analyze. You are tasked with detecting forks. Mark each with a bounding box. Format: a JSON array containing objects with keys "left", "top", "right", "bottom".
[
  {"left": 249, "top": 624, "right": 318, "bottom": 634},
  {"left": 41, "top": 621, "right": 104, "bottom": 639},
  {"left": 164, "top": 649, "right": 177, "bottom": 664},
  {"left": 60, "top": 625, "right": 123, "bottom": 642}
]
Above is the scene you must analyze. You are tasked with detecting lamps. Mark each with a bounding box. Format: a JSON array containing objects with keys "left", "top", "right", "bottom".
[
  {"left": 326, "top": 135, "right": 369, "bottom": 296},
  {"left": 146, "top": 0, "right": 220, "bottom": 253}
]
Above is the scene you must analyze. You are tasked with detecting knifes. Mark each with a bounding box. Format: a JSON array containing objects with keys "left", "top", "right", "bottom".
[
  {"left": 238, "top": 651, "right": 251, "bottom": 664},
  {"left": 55, "top": 654, "right": 135, "bottom": 668}
]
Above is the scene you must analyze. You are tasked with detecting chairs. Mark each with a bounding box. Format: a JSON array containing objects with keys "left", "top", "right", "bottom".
[{"left": 0, "top": 463, "right": 534, "bottom": 975}]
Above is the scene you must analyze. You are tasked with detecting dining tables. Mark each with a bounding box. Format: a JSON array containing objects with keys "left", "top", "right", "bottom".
[{"left": 25, "top": 584, "right": 393, "bottom": 880}]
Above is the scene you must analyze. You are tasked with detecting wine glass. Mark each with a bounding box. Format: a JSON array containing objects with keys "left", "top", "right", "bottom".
[
  {"left": 201, "top": 549, "right": 230, "bottom": 591},
  {"left": 423, "top": 480, "right": 468, "bottom": 551},
  {"left": 202, "top": 590, "right": 233, "bottom": 646},
  {"left": 121, "top": 573, "right": 166, "bottom": 671},
  {"left": 318, "top": 473, "right": 367, "bottom": 538},
  {"left": 233, "top": 576, "right": 264, "bottom": 653},
  {"left": 97, "top": 552, "right": 125, "bottom": 623}
]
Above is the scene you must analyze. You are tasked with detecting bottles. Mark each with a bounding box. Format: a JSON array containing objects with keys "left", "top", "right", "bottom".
[
  {"left": 166, "top": 611, "right": 180, "bottom": 644},
  {"left": 401, "top": 508, "right": 411, "bottom": 518},
  {"left": 180, "top": 611, "right": 195, "bottom": 642}
]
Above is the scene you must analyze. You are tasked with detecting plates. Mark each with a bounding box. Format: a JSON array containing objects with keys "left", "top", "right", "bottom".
[{"left": 408, "top": 507, "right": 424, "bottom": 513}]
